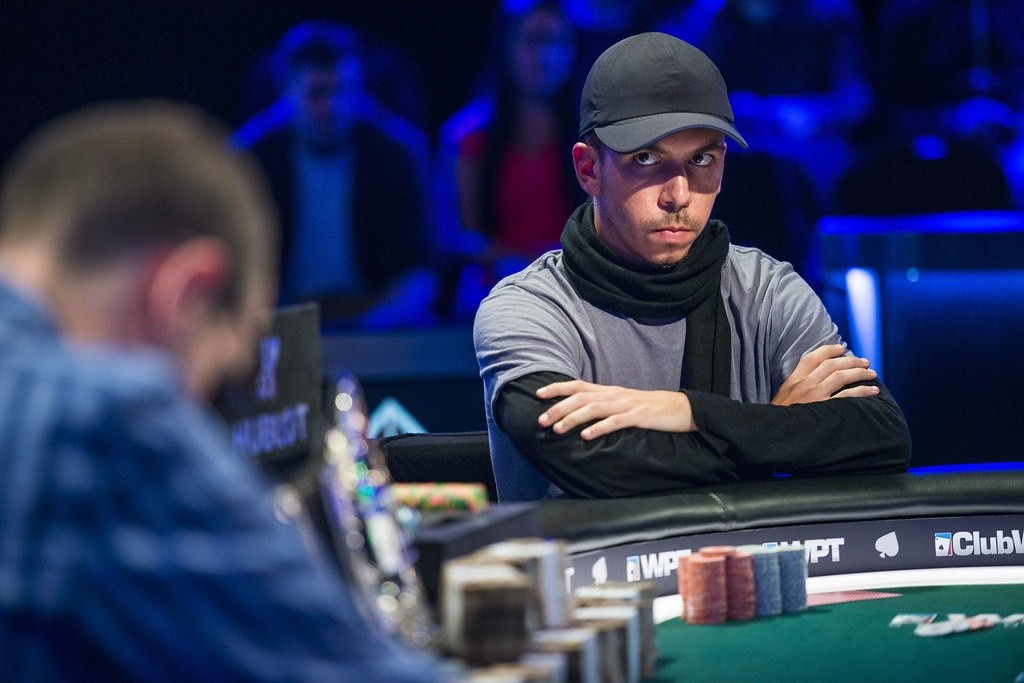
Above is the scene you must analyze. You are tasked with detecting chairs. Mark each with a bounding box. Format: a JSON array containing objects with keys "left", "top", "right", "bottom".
[{"left": 213, "top": 209, "right": 1024, "bottom": 683}]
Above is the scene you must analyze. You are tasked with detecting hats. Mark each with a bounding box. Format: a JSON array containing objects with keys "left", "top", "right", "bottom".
[{"left": 579, "top": 31, "right": 749, "bottom": 153}]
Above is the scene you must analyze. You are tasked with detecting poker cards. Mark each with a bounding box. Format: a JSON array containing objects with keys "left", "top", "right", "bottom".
[{"left": 805, "top": 589, "right": 905, "bottom": 609}]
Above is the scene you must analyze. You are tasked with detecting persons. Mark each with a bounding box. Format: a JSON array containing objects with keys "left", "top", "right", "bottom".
[
  {"left": 427, "top": 0, "right": 589, "bottom": 325},
  {"left": 473, "top": 31, "right": 912, "bottom": 504},
  {"left": 0, "top": 106, "right": 468, "bottom": 683},
  {"left": 231, "top": 22, "right": 436, "bottom": 331}
]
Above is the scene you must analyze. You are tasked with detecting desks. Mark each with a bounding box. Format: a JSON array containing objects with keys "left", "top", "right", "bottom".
[{"left": 653, "top": 585, "right": 1024, "bottom": 683}]
